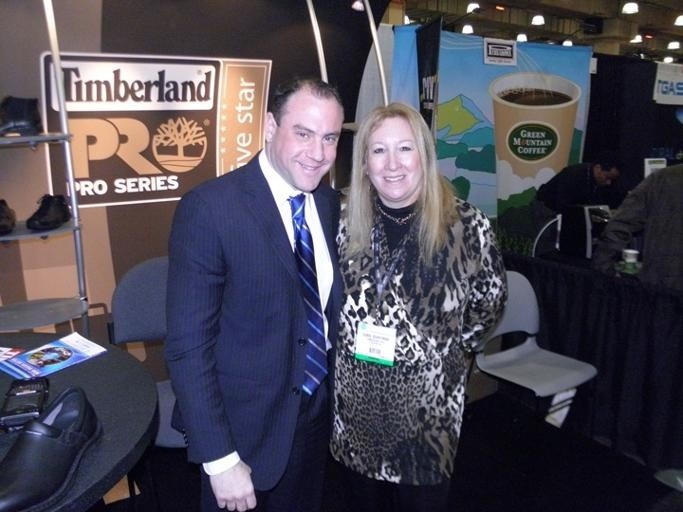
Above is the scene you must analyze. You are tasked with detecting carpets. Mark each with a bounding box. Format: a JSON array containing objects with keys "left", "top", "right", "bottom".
[{"left": 101, "top": 390, "right": 683, "bottom": 512}]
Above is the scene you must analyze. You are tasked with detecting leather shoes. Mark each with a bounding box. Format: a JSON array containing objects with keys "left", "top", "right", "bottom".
[
  {"left": 0, "top": 96, "right": 43, "bottom": 136},
  {"left": 0, "top": 199, "right": 15, "bottom": 235},
  {"left": 27, "top": 194, "right": 71, "bottom": 229},
  {"left": 0, "top": 387, "right": 101, "bottom": 512}
]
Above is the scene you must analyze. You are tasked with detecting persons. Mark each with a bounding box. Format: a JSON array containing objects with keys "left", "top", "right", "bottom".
[
  {"left": 528, "top": 155, "right": 622, "bottom": 242},
  {"left": 164, "top": 75, "right": 346, "bottom": 512},
  {"left": 594, "top": 163, "right": 681, "bottom": 293},
  {"left": 330, "top": 100, "right": 506, "bottom": 510}
]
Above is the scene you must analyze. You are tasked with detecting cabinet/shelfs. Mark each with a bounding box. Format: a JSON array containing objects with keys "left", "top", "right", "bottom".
[{"left": 0, "top": 0, "right": 91, "bottom": 343}]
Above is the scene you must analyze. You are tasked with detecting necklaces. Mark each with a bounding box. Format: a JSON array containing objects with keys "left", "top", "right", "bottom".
[{"left": 376, "top": 205, "right": 418, "bottom": 228}]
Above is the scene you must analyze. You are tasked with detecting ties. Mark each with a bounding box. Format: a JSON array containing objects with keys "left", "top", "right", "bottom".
[{"left": 287, "top": 193, "right": 328, "bottom": 395}]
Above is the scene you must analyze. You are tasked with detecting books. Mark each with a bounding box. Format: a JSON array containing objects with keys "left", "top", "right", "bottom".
[{"left": 0, "top": 332, "right": 107, "bottom": 380}]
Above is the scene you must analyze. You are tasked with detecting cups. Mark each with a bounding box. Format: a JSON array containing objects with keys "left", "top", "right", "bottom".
[
  {"left": 622, "top": 248, "right": 640, "bottom": 273},
  {"left": 488, "top": 69, "right": 584, "bottom": 220}
]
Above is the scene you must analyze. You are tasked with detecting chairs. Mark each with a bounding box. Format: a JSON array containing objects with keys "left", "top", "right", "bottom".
[
  {"left": 465, "top": 270, "right": 598, "bottom": 499},
  {"left": 108, "top": 256, "right": 202, "bottom": 510}
]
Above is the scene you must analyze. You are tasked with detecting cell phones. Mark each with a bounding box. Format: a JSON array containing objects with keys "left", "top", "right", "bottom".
[{"left": 0, "top": 377, "right": 49, "bottom": 432}]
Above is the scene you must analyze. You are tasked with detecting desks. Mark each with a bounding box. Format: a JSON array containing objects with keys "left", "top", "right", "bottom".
[{"left": 0, "top": 333, "right": 160, "bottom": 511}]
